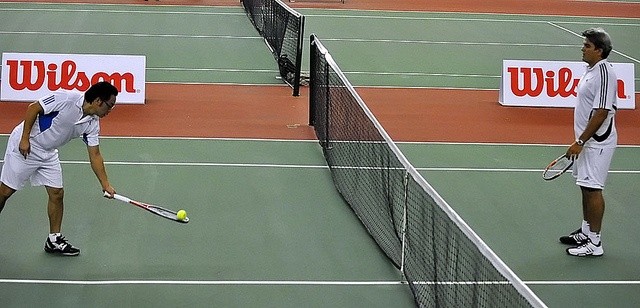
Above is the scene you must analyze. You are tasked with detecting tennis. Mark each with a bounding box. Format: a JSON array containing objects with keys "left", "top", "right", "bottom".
[{"left": 177, "top": 209, "right": 187, "bottom": 220}]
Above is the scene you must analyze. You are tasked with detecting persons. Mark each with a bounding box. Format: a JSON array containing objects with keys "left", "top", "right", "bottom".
[
  {"left": 0, "top": 81, "right": 118, "bottom": 256},
  {"left": 559, "top": 27, "right": 619, "bottom": 257}
]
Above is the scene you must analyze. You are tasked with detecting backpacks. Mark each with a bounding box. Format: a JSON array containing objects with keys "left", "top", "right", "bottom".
[{"left": 278, "top": 53, "right": 311, "bottom": 87}]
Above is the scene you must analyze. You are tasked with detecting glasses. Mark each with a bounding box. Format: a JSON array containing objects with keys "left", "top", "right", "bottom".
[{"left": 104, "top": 101, "right": 116, "bottom": 110}]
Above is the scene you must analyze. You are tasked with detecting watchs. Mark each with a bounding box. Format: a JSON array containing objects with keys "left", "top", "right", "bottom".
[{"left": 575, "top": 139, "right": 584, "bottom": 146}]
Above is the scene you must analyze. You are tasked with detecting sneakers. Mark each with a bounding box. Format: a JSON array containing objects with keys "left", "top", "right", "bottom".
[
  {"left": 565, "top": 238, "right": 604, "bottom": 257},
  {"left": 559, "top": 228, "right": 588, "bottom": 245},
  {"left": 43, "top": 235, "right": 80, "bottom": 256}
]
